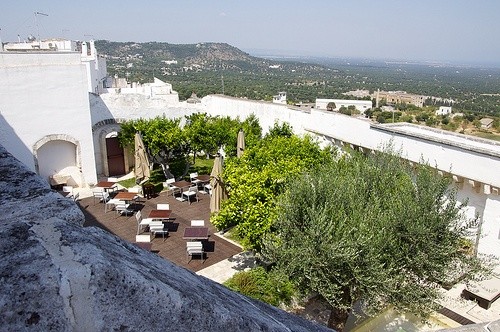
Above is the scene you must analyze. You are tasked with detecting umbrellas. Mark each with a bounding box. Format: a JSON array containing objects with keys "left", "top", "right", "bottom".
[
  {"left": 210, "top": 153, "right": 230, "bottom": 216},
  {"left": 236, "top": 127, "right": 246, "bottom": 159},
  {"left": 134, "top": 129, "right": 151, "bottom": 188}
]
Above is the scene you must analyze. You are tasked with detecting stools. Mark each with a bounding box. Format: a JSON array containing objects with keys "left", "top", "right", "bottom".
[{"left": 143, "top": 184, "right": 156, "bottom": 200}]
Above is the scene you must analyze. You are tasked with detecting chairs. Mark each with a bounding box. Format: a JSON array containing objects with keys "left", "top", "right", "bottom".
[
  {"left": 63, "top": 186, "right": 75, "bottom": 202},
  {"left": 104, "top": 178, "right": 118, "bottom": 192},
  {"left": 104, "top": 192, "right": 117, "bottom": 213},
  {"left": 190, "top": 172, "right": 202, "bottom": 192},
  {"left": 166, "top": 178, "right": 181, "bottom": 198},
  {"left": 204, "top": 184, "right": 212, "bottom": 194},
  {"left": 191, "top": 220, "right": 204, "bottom": 228},
  {"left": 114, "top": 200, "right": 131, "bottom": 220},
  {"left": 92, "top": 187, "right": 106, "bottom": 205},
  {"left": 136, "top": 235, "right": 150, "bottom": 242},
  {"left": 150, "top": 220, "right": 168, "bottom": 242},
  {"left": 157, "top": 204, "right": 170, "bottom": 220},
  {"left": 182, "top": 185, "right": 199, "bottom": 205},
  {"left": 127, "top": 188, "right": 140, "bottom": 209},
  {"left": 136, "top": 210, "right": 152, "bottom": 235},
  {"left": 186, "top": 242, "right": 204, "bottom": 264}
]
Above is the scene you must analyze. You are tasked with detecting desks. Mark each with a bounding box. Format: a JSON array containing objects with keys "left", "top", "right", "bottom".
[
  {"left": 132, "top": 242, "right": 151, "bottom": 251},
  {"left": 148, "top": 209, "right": 172, "bottom": 229},
  {"left": 58, "top": 191, "right": 70, "bottom": 198},
  {"left": 112, "top": 191, "right": 138, "bottom": 210},
  {"left": 183, "top": 227, "right": 208, "bottom": 244},
  {"left": 195, "top": 175, "right": 212, "bottom": 194},
  {"left": 170, "top": 180, "right": 194, "bottom": 202},
  {"left": 95, "top": 182, "right": 116, "bottom": 200}
]
